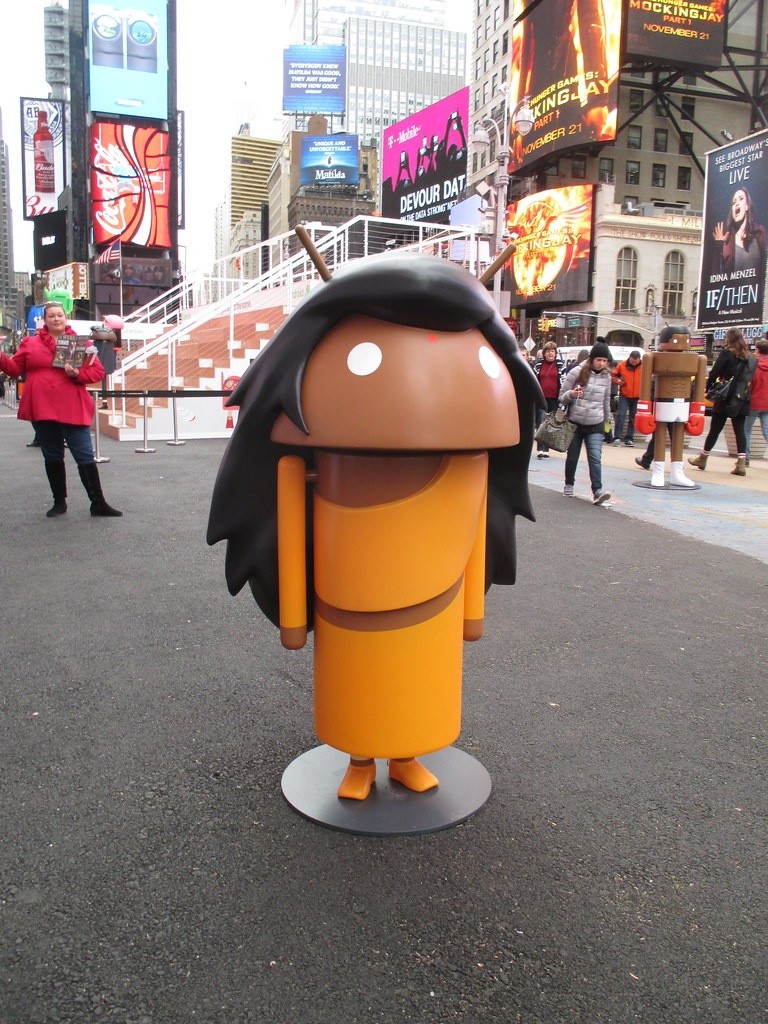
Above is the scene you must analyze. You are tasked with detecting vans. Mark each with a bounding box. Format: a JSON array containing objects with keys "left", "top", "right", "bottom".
[{"left": 536, "top": 346, "right": 646, "bottom": 396}]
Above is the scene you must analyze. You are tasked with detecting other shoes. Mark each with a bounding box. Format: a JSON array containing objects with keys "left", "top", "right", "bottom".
[{"left": 537, "top": 451, "right": 550, "bottom": 460}]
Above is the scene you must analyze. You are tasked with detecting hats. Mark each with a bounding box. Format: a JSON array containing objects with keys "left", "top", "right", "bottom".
[{"left": 590, "top": 342, "right": 610, "bottom": 358}]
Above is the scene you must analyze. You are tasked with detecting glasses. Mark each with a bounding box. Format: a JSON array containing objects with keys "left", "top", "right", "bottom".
[
  {"left": 44, "top": 301, "right": 63, "bottom": 308},
  {"left": 630, "top": 361, "right": 639, "bottom": 364}
]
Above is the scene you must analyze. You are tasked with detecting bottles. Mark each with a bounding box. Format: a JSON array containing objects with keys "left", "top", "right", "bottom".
[{"left": 34, "top": 111, "right": 55, "bottom": 193}]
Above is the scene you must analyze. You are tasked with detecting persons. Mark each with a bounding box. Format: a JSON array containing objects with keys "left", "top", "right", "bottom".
[
  {"left": 394, "top": 116, "right": 467, "bottom": 189},
  {"left": 712, "top": 187, "right": 765, "bottom": 319},
  {"left": 0, "top": 302, "right": 125, "bottom": 517},
  {"left": 205, "top": 230, "right": 547, "bottom": 801},
  {"left": 634, "top": 322, "right": 706, "bottom": 488},
  {"left": 522, "top": 327, "right": 768, "bottom": 506}
]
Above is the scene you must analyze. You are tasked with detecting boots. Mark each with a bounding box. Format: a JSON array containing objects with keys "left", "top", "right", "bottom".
[
  {"left": 44, "top": 461, "right": 67, "bottom": 517},
  {"left": 688, "top": 453, "right": 709, "bottom": 470},
  {"left": 77, "top": 462, "right": 123, "bottom": 517},
  {"left": 730, "top": 457, "right": 746, "bottom": 476}
]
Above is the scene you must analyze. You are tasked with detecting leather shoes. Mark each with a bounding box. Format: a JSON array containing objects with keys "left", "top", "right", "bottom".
[
  {"left": 26, "top": 440, "right": 41, "bottom": 447},
  {"left": 635, "top": 456, "right": 649, "bottom": 469}
]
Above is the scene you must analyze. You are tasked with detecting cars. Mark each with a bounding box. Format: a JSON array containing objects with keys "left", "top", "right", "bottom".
[{"left": 690, "top": 375, "right": 714, "bottom": 412}]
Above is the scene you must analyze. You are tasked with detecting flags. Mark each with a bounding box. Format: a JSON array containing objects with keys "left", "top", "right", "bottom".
[{"left": 92, "top": 240, "right": 121, "bottom": 264}]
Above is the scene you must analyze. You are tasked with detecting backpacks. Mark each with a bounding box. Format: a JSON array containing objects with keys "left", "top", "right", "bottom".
[{"left": 720, "top": 351, "right": 759, "bottom": 418}]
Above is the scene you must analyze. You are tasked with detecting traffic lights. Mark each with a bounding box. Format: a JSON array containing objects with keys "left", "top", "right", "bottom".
[{"left": 537, "top": 319, "right": 545, "bottom": 331}]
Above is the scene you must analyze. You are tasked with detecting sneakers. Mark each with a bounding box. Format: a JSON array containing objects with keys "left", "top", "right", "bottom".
[
  {"left": 735, "top": 459, "right": 749, "bottom": 467},
  {"left": 593, "top": 489, "right": 611, "bottom": 506},
  {"left": 623, "top": 440, "right": 635, "bottom": 447},
  {"left": 613, "top": 439, "right": 621, "bottom": 447},
  {"left": 562, "top": 484, "right": 576, "bottom": 498}
]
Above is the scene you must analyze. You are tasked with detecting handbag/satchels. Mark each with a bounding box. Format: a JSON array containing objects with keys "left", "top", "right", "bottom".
[
  {"left": 533, "top": 399, "right": 578, "bottom": 453},
  {"left": 704, "top": 379, "right": 732, "bottom": 403}
]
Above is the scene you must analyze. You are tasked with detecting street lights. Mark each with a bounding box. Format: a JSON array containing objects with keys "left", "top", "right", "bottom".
[{"left": 472, "top": 95, "right": 534, "bottom": 312}]
